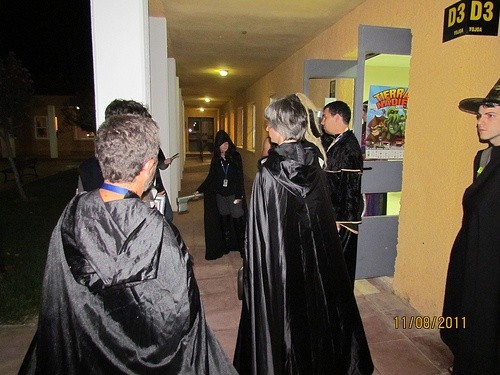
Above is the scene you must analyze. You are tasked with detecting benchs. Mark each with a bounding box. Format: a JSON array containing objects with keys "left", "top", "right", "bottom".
[{"left": 0, "top": 153, "right": 39, "bottom": 183}]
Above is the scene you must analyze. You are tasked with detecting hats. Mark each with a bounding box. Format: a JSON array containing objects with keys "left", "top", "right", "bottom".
[{"left": 458, "top": 77, "right": 500, "bottom": 113}]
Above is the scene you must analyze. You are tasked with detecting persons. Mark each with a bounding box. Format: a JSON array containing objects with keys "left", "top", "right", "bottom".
[
  {"left": 440, "top": 78, "right": 500, "bottom": 375},
  {"left": 16, "top": 92, "right": 375, "bottom": 375}
]
